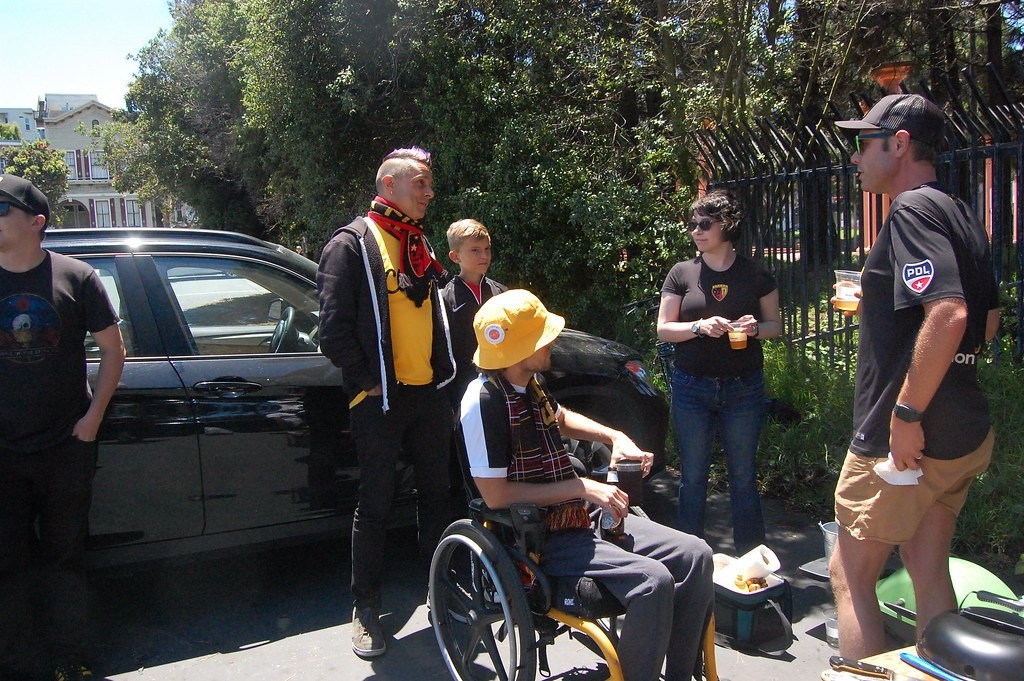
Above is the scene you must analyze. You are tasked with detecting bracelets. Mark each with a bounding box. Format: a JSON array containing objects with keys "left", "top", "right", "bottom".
[{"left": 753, "top": 326, "right": 759, "bottom": 337}]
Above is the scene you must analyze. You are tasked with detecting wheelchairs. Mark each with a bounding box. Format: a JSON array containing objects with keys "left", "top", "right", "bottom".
[{"left": 428, "top": 407, "right": 721, "bottom": 681}]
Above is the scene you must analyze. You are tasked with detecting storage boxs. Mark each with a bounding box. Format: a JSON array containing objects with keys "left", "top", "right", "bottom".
[{"left": 713, "top": 553, "right": 793, "bottom": 650}]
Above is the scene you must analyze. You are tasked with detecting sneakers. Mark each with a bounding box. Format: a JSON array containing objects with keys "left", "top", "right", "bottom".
[
  {"left": 54, "top": 660, "right": 95, "bottom": 681},
  {"left": 350, "top": 605, "right": 387, "bottom": 655},
  {"left": 426, "top": 583, "right": 480, "bottom": 623}
]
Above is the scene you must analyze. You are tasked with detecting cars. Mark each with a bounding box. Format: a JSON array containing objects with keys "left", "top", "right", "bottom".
[{"left": 34, "top": 226, "right": 674, "bottom": 573}]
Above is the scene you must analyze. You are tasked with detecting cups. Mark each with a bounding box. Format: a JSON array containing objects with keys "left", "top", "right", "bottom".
[
  {"left": 616, "top": 462, "right": 644, "bottom": 504},
  {"left": 728, "top": 320, "right": 747, "bottom": 349},
  {"left": 835, "top": 270, "right": 861, "bottom": 311},
  {"left": 824, "top": 609, "right": 838, "bottom": 648}
]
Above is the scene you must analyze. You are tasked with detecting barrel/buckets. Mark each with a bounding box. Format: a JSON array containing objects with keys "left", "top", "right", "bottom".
[{"left": 819, "top": 520, "right": 839, "bottom": 564}]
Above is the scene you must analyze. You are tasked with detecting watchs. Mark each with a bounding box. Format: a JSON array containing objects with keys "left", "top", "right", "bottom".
[
  {"left": 894, "top": 403, "right": 923, "bottom": 423},
  {"left": 692, "top": 324, "right": 705, "bottom": 338}
]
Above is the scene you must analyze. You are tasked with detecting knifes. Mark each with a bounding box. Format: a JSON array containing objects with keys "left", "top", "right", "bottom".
[{"left": 830, "top": 656, "right": 925, "bottom": 681}]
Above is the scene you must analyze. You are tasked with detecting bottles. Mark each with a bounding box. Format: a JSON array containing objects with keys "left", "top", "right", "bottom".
[{"left": 601, "top": 466, "right": 625, "bottom": 541}]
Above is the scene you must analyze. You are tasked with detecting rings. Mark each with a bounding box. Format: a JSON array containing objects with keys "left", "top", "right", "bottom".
[
  {"left": 720, "top": 326, "right": 723, "bottom": 330},
  {"left": 917, "top": 455, "right": 922, "bottom": 459}
]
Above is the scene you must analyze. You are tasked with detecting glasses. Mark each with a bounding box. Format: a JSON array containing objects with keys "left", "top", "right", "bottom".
[
  {"left": 0, "top": 200, "right": 38, "bottom": 217},
  {"left": 855, "top": 132, "right": 898, "bottom": 155},
  {"left": 687, "top": 218, "right": 726, "bottom": 232}
]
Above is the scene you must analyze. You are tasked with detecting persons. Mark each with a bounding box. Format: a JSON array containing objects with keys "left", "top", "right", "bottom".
[
  {"left": 296, "top": 246, "right": 304, "bottom": 256},
  {"left": 454, "top": 289, "right": 715, "bottom": 681},
  {"left": 315, "top": 146, "right": 470, "bottom": 656},
  {"left": 0, "top": 173, "right": 127, "bottom": 681},
  {"left": 443, "top": 219, "right": 508, "bottom": 401},
  {"left": 828, "top": 93, "right": 1001, "bottom": 659},
  {"left": 658, "top": 189, "right": 782, "bottom": 557}
]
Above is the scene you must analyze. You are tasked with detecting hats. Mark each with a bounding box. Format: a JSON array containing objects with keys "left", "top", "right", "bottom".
[
  {"left": 833, "top": 94, "right": 945, "bottom": 148},
  {"left": 472, "top": 288, "right": 565, "bottom": 369},
  {"left": 0, "top": 173, "right": 51, "bottom": 229}
]
